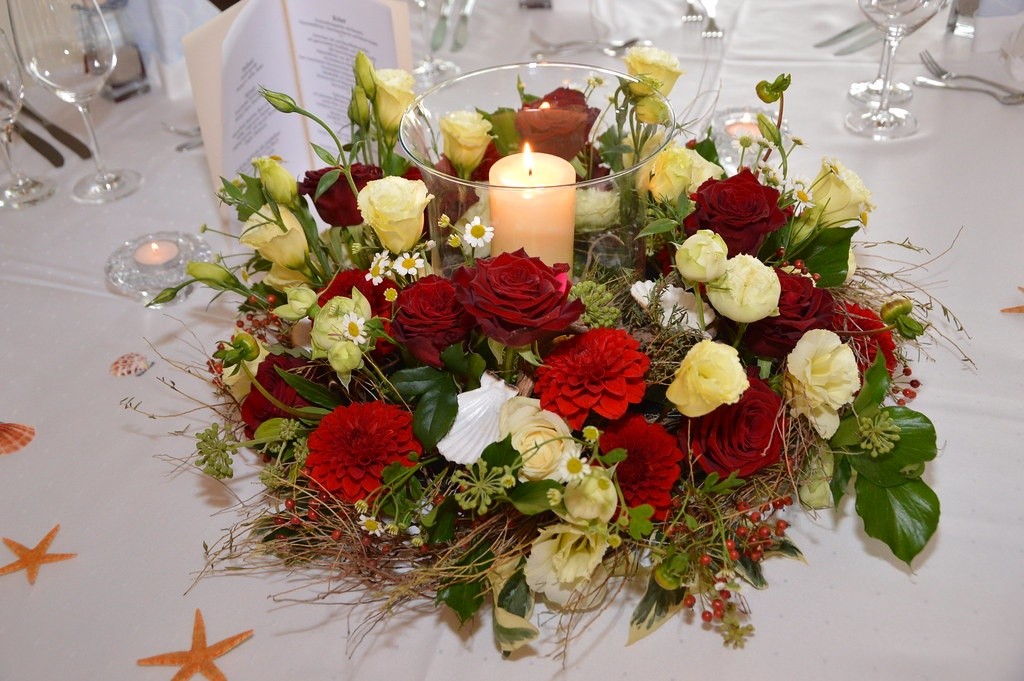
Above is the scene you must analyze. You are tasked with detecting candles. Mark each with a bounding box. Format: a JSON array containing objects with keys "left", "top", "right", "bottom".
[{"left": 488, "top": 139, "right": 576, "bottom": 285}]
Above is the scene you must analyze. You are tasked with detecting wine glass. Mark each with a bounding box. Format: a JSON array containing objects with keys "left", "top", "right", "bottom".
[
  {"left": 845, "top": 1, "right": 954, "bottom": 102},
  {"left": 410, "top": 0, "right": 460, "bottom": 95},
  {"left": 0, "top": 29, "right": 55, "bottom": 210},
  {"left": 3, "top": 0, "right": 145, "bottom": 204},
  {"left": 842, "top": 0, "right": 946, "bottom": 138}
]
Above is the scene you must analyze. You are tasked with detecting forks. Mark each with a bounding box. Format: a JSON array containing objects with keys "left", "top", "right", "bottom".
[
  {"left": 681, "top": 0, "right": 723, "bottom": 56},
  {"left": 919, "top": 49, "right": 1024, "bottom": 98},
  {"left": 912, "top": 74, "right": 1024, "bottom": 107}
]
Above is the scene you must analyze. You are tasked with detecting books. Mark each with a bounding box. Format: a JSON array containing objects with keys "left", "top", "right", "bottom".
[{"left": 183, "top": 2, "right": 414, "bottom": 247}]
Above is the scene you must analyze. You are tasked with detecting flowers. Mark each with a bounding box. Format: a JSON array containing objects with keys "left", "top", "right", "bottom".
[{"left": 117, "top": 41, "right": 973, "bottom": 678}]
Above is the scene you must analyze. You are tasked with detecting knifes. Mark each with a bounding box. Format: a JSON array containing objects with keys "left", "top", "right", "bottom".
[
  {"left": 0, "top": 80, "right": 93, "bottom": 160},
  {"left": 430, "top": 0, "right": 454, "bottom": 52},
  {"left": 944, "top": 2, "right": 966, "bottom": 32},
  {"left": 0, "top": 99, "right": 65, "bottom": 169},
  {"left": 815, "top": 16, "right": 885, "bottom": 48},
  {"left": 834, "top": 28, "right": 908, "bottom": 58},
  {"left": 451, "top": 0, "right": 475, "bottom": 52}
]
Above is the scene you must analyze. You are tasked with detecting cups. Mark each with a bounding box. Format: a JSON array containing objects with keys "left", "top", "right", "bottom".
[{"left": 398, "top": 60, "right": 676, "bottom": 347}]
